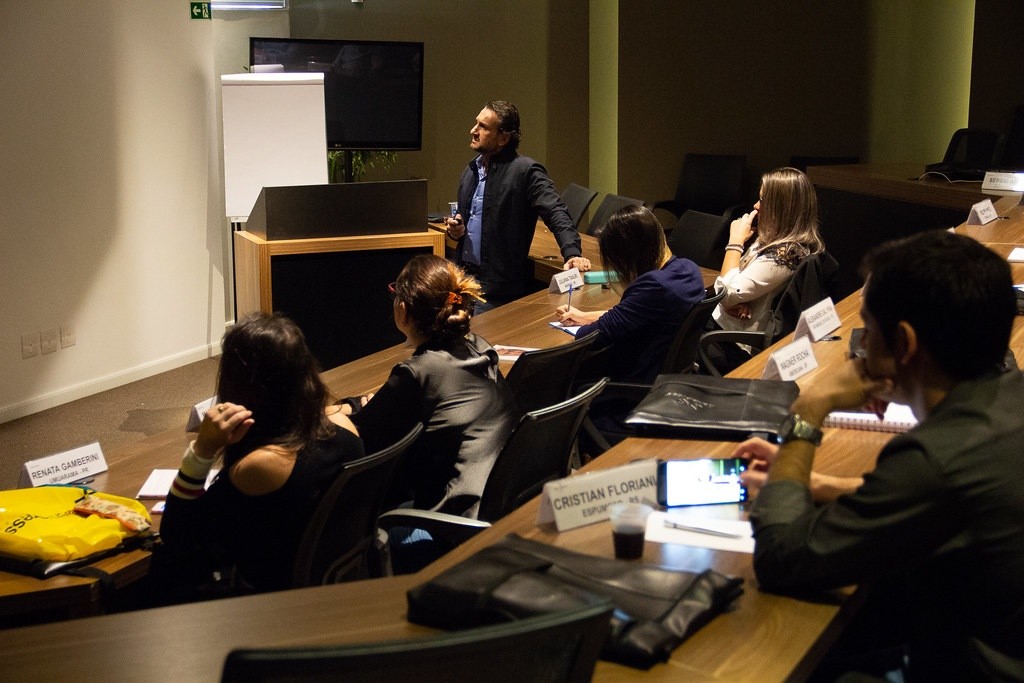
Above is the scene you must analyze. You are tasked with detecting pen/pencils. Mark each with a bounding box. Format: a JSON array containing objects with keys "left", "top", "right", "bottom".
[
  {"left": 568, "top": 282, "right": 573, "bottom": 311},
  {"left": 666, "top": 520, "right": 746, "bottom": 541}
]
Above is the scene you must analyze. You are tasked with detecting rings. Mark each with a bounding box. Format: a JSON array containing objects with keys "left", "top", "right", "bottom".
[{"left": 584, "top": 265, "right": 588, "bottom": 267}]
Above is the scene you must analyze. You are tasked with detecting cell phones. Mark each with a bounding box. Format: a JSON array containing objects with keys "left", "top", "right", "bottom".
[{"left": 656, "top": 457, "right": 750, "bottom": 507}]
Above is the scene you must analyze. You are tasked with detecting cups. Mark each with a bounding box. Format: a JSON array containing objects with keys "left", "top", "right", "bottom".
[
  {"left": 607, "top": 503, "right": 652, "bottom": 560},
  {"left": 448, "top": 202, "right": 458, "bottom": 219}
]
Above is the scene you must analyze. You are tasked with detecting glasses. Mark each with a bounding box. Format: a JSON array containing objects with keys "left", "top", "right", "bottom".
[{"left": 387, "top": 282, "right": 415, "bottom": 315}]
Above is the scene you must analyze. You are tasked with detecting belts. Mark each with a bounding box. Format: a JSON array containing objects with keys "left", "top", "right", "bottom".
[{"left": 460, "top": 262, "right": 481, "bottom": 275}]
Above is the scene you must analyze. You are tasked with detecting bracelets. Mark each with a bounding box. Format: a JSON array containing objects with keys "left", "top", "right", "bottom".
[{"left": 725, "top": 243, "right": 744, "bottom": 254}]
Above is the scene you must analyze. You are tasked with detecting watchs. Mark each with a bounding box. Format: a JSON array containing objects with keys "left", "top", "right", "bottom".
[{"left": 777, "top": 412, "right": 824, "bottom": 447}]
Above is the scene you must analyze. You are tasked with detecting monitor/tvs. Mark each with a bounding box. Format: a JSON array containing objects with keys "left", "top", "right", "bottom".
[{"left": 249, "top": 37, "right": 423, "bottom": 151}]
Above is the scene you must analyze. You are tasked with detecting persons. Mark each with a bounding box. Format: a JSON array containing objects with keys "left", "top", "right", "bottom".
[
  {"left": 159, "top": 310, "right": 365, "bottom": 607},
  {"left": 325, "top": 254, "right": 523, "bottom": 576},
  {"left": 555, "top": 204, "right": 704, "bottom": 471},
  {"left": 447, "top": 99, "right": 592, "bottom": 318},
  {"left": 729, "top": 230, "right": 1024, "bottom": 683},
  {"left": 694, "top": 168, "right": 823, "bottom": 377}
]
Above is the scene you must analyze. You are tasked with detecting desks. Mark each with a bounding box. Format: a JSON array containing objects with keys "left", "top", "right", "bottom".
[{"left": 0, "top": 163, "right": 1024, "bottom": 683}]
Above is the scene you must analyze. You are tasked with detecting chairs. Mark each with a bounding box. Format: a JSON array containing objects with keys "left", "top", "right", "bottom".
[
  {"left": 221, "top": 600, "right": 616, "bottom": 683},
  {"left": 283, "top": 153, "right": 870, "bottom": 587},
  {"left": 944, "top": 128, "right": 1006, "bottom": 168}
]
